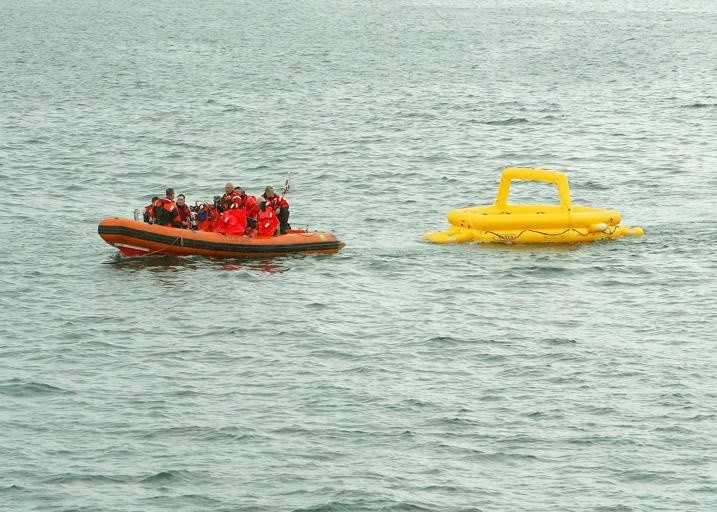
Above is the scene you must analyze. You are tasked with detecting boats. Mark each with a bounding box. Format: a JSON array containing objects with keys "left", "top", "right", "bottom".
[
  {"left": 420, "top": 164, "right": 650, "bottom": 247},
  {"left": 91, "top": 214, "right": 346, "bottom": 264}
]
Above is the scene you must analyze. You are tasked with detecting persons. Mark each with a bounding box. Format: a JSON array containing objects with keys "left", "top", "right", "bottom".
[{"left": 143, "top": 182, "right": 291, "bottom": 236}]
